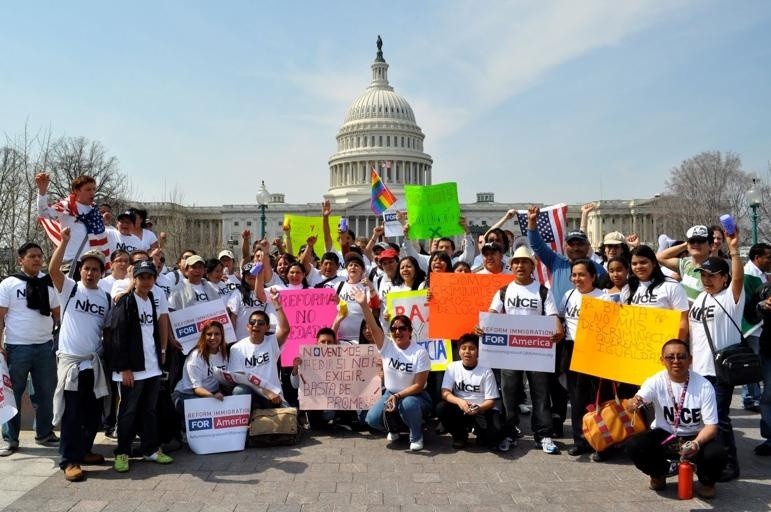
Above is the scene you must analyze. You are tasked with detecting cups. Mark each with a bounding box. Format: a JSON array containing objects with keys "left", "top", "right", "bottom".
[
  {"left": 663, "top": 353, "right": 689, "bottom": 360},
  {"left": 249, "top": 317, "right": 266, "bottom": 326},
  {"left": 390, "top": 326, "right": 409, "bottom": 333}
]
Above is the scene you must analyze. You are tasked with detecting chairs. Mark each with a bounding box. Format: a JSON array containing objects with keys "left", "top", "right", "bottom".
[
  {"left": 514, "top": 425, "right": 524, "bottom": 437},
  {"left": 699, "top": 481, "right": 717, "bottom": 498},
  {"left": 114, "top": 453, "right": 131, "bottom": 472},
  {"left": 79, "top": 451, "right": 105, "bottom": 465},
  {"left": 453, "top": 437, "right": 518, "bottom": 452},
  {"left": 387, "top": 431, "right": 402, "bottom": 441},
  {"left": 536, "top": 436, "right": 560, "bottom": 454},
  {"left": 104, "top": 432, "right": 120, "bottom": 442},
  {"left": 719, "top": 470, "right": 741, "bottom": 481},
  {"left": 410, "top": 434, "right": 424, "bottom": 451},
  {"left": 0, "top": 439, "right": 19, "bottom": 457},
  {"left": 649, "top": 473, "right": 666, "bottom": 490},
  {"left": 520, "top": 401, "right": 531, "bottom": 415},
  {"left": 754, "top": 438, "right": 771, "bottom": 456},
  {"left": 34, "top": 431, "right": 63, "bottom": 447},
  {"left": 62, "top": 461, "right": 87, "bottom": 482},
  {"left": 568, "top": 442, "right": 585, "bottom": 457},
  {"left": 143, "top": 450, "right": 174, "bottom": 465}
]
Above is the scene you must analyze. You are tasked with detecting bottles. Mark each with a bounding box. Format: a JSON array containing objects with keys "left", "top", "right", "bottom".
[
  {"left": 604, "top": 231, "right": 627, "bottom": 246},
  {"left": 264, "top": 240, "right": 393, "bottom": 260},
  {"left": 694, "top": 258, "right": 730, "bottom": 276},
  {"left": 118, "top": 210, "right": 136, "bottom": 225},
  {"left": 482, "top": 242, "right": 503, "bottom": 254},
  {"left": 134, "top": 262, "right": 157, "bottom": 279},
  {"left": 686, "top": 225, "right": 714, "bottom": 244},
  {"left": 378, "top": 248, "right": 399, "bottom": 265},
  {"left": 566, "top": 229, "right": 588, "bottom": 243},
  {"left": 81, "top": 249, "right": 107, "bottom": 266},
  {"left": 508, "top": 246, "right": 538, "bottom": 270},
  {"left": 219, "top": 250, "right": 235, "bottom": 261},
  {"left": 185, "top": 254, "right": 206, "bottom": 269}
]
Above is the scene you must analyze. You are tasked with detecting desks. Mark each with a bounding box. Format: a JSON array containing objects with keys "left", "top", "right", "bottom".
[
  {"left": 254, "top": 180, "right": 272, "bottom": 242},
  {"left": 747, "top": 177, "right": 762, "bottom": 247},
  {"left": 628, "top": 200, "right": 642, "bottom": 236}
]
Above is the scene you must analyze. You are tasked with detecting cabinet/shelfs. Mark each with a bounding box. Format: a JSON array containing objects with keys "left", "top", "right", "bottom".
[
  {"left": 340, "top": 301, "right": 349, "bottom": 316},
  {"left": 720, "top": 214, "right": 737, "bottom": 235},
  {"left": 249, "top": 263, "right": 263, "bottom": 276},
  {"left": 341, "top": 216, "right": 347, "bottom": 232},
  {"left": 677, "top": 455, "right": 695, "bottom": 500}
]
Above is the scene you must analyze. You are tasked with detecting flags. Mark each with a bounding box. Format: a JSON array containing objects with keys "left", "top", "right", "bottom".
[
  {"left": 37, "top": 194, "right": 111, "bottom": 270},
  {"left": 370, "top": 173, "right": 398, "bottom": 217},
  {"left": 516, "top": 203, "right": 568, "bottom": 289}
]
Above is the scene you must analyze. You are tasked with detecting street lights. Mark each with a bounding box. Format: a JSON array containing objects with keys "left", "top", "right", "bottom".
[
  {"left": 712, "top": 340, "right": 764, "bottom": 385},
  {"left": 249, "top": 407, "right": 300, "bottom": 447},
  {"left": 582, "top": 377, "right": 649, "bottom": 451}
]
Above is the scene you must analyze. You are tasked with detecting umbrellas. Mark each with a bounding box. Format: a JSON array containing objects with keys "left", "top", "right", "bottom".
[{"left": 593, "top": 452, "right": 612, "bottom": 461}]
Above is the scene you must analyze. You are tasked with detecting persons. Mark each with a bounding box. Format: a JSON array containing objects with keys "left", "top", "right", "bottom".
[
  {"left": 527, "top": 206, "right": 770, "bottom": 483},
  {"left": 171, "top": 321, "right": 228, "bottom": 444},
  {"left": 228, "top": 288, "right": 291, "bottom": 448},
  {"left": 48, "top": 227, "right": 114, "bottom": 482},
  {"left": 436, "top": 333, "right": 506, "bottom": 451},
  {"left": 35, "top": 173, "right": 172, "bottom": 282},
  {"left": 96, "top": 248, "right": 175, "bottom": 472},
  {"left": 2, "top": 243, "right": 60, "bottom": 456},
  {"left": 166, "top": 199, "right": 564, "bottom": 447},
  {"left": 472, "top": 245, "right": 563, "bottom": 454},
  {"left": 625, "top": 339, "right": 728, "bottom": 498},
  {"left": 349, "top": 286, "right": 430, "bottom": 451}
]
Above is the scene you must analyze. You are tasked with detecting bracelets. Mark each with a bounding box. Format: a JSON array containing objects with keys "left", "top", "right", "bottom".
[
  {"left": 161, "top": 348, "right": 166, "bottom": 353},
  {"left": 730, "top": 251, "right": 740, "bottom": 256},
  {"left": 275, "top": 306, "right": 282, "bottom": 311},
  {"left": 394, "top": 392, "right": 401, "bottom": 401}
]
